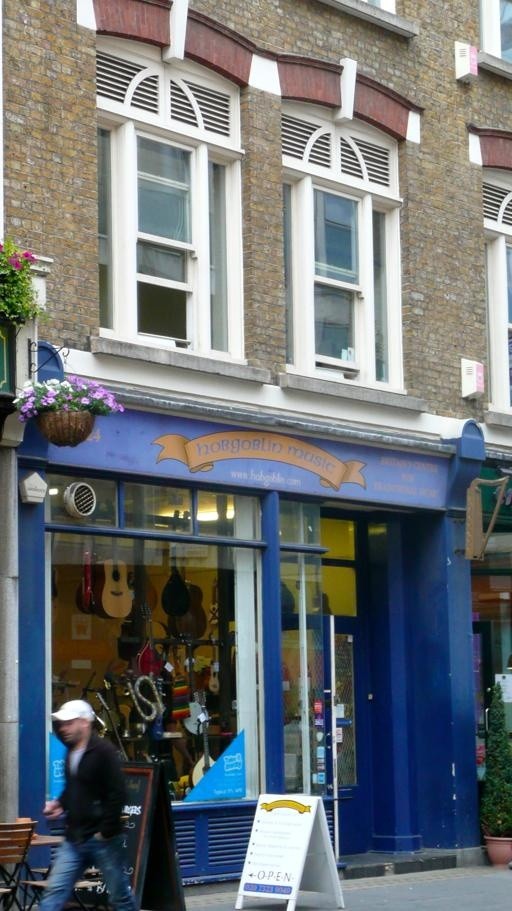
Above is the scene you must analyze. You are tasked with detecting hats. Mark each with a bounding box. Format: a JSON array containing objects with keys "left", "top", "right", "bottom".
[{"left": 52, "top": 699, "right": 95, "bottom": 722}]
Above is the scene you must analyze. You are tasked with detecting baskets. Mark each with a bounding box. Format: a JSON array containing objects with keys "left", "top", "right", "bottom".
[{"left": 34, "top": 410, "right": 95, "bottom": 447}]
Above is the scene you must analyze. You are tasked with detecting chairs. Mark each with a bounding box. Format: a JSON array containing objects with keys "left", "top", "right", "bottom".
[{"left": 0, "top": 818, "right": 40, "bottom": 911}]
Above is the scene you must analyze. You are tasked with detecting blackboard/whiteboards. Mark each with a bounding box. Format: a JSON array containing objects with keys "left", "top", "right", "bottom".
[{"left": 69, "top": 768, "right": 155, "bottom": 896}]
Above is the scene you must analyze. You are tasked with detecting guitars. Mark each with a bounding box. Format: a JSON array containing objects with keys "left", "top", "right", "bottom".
[{"left": 76, "top": 499, "right": 220, "bottom": 697}]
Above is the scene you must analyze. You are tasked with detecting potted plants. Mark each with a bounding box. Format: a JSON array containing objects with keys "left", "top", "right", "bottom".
[{"left": 478, "top": 680, "right": 511, "bottom": 872}]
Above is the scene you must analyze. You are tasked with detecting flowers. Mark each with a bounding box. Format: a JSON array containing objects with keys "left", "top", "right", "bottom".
[
  {"left": 1, "top": 238, "right": 55, "bottom": 329},
  {"left": 10, "top": 375, "right": 126, "bottom": 425}
]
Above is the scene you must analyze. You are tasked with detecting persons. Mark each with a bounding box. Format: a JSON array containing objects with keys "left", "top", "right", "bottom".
[{"left": 37, "top": 700, "right": 140, "bottom": 911}]
